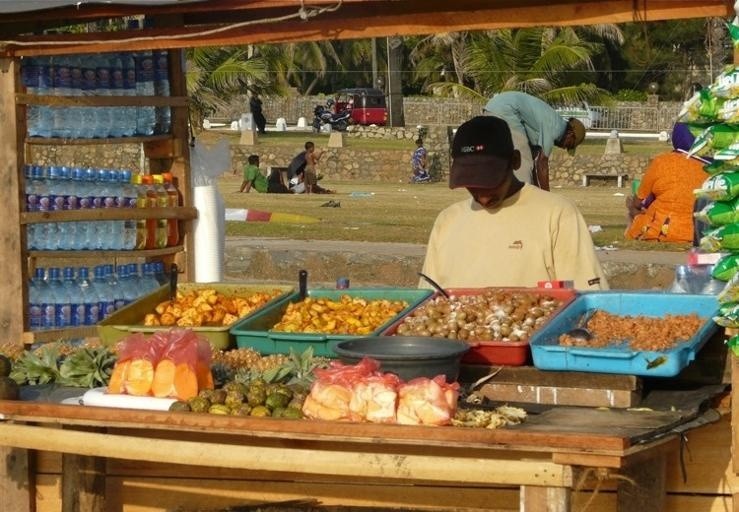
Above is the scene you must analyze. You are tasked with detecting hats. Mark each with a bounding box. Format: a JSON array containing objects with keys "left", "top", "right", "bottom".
[
  {"left": 672, "top": 123, "right": 696, "bottom": 150},
  {"left": 569, "top": 117, "right": 586, "bottom": 159},
  {"left": 449, "top": 116, "right": 515, "bottom": 192}
]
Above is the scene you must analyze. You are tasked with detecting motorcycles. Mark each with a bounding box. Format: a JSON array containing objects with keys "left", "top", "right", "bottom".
[
  {"left": 312, "top": 105, "right": 350, "bottom": 131},
  {"left": 334, "top": 88, "right": 388, "bottom": 126},
  {"left": 552, "top": 92, "right": 607, "bottom": 129}
]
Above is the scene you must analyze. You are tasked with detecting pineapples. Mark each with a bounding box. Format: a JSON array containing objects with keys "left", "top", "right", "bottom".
[
  {"left": 0, "top": 337, "right": 124, "bottom": 389},
  {"left": 202, "top": 343, "right": 333, "bottom": 385}
]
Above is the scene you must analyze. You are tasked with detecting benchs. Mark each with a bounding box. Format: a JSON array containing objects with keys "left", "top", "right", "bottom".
[{"left": 582, "top": 172, "right": 627, "bottom": 187}]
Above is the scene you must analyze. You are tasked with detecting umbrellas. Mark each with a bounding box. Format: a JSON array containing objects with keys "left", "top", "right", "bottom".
[{"left": 288, "top": 151, "right": 307, "bottom": 179}]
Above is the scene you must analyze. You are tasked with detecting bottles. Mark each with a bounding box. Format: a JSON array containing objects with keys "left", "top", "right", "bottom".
[
  {"left": 28, "top": 262, "right": 165, "bottom": 330},
  {"left": 22, "top": 163, "right": 181, "bottom": 249},
  {"left": 22, "top": 51, "right": 172, "bottom": 136}
]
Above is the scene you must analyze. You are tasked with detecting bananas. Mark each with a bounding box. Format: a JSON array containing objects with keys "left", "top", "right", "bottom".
[{"left": 304, "top": 381, "right": 361, "bottom": 424}]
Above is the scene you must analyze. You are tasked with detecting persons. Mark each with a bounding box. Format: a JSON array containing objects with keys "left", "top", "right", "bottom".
[
  {"left": 250, "top": 91, "right": 266, "bottom": 132},
  {"left": 240, "top": 155, "right": 294, "bottom": 193},
  {"left": 288, "top": 170, "right": 307, "bottom": 194},
  {"left": 417, "top": 115, "right": 611, "bottom": 292},
  {"left": 413, "top": 138, "right": 430, "bottom": 181},
  {"left": 483, "top": 90, "right": 586, "bottom": 192},
  {"left": 623, "top": 122, "right": 723, "bottom": 242},
  {"left": 304, "top": 142, "right": 326, "bottom": 193}
]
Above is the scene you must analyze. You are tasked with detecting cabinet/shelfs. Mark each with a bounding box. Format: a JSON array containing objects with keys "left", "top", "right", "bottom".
[
  {"left": 0, "top": 324, "right": 739, "bottom": 511},
  {"left": 0, "top": 47, "right": 197, "bottom": 350}
]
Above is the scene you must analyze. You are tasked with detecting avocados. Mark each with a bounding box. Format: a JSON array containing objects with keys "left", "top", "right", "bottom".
[
  {"left": 169, "top": 377, "right": 315, "bottom": 418},
  {"left": 0, "top": 355, "right": 20, "bottom": 399}
]
